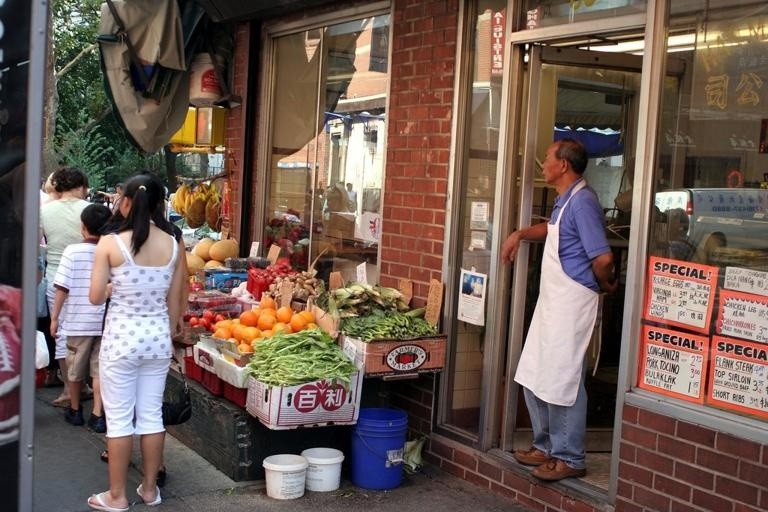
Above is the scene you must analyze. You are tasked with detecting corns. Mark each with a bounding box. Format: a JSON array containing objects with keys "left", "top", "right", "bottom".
[{"left": 315, "top": 282, "right": 403, "bottom": 318}]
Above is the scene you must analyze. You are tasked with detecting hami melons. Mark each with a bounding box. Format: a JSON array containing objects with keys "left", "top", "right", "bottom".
[{"left": 185, "top": 237, "right": 239, "bottom": 275}]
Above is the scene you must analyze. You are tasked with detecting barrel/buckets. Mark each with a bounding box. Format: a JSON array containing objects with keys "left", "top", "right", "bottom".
[
  {"left": 301, "top": 448, "right": 344, "bottom": 492},
  {"left": 263, "top": 454, "right": 308, "bottom": 499},
  {"left": 353, "top": 408, "right": 409, "bottom": 490}
]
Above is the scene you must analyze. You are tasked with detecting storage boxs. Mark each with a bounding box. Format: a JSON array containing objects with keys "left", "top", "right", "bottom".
[
  {"left": 338, "top": 334, "right": 448, "bottom": 378},
  {"left": 204, "top": 268, "right": 249, "bottom": 293},
  {"left": 184, "top": 356, "right": 249, "bottom": 408},
  {"left": 216, "top": 354, "right": 252, "bottom": 389},
  {"left": 244, "top": 351, "right": 364, "bottom": 430},
  {"left": 246, "top": 266, "right": 305, "bottom": 302},
  {"left": 183, "top": 296, "right": 242, "bottom": 329},
  {"left": 216, "top": 340, "right": 252, "bottom": 388},
  {"left": 224, "top": 257, "right": 271, "bottom": 269},
  {"left": 312, "top": 303, "right": 358, "bottom": 339},
  {"left": 193, "top": 341, "right": 222, "bottom": 373}
]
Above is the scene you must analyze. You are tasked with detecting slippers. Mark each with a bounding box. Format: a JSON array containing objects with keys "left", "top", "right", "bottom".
[
  {"left": 84, "top": 495, "right": 126, "bottom": 510},
  {"left": 133, "top": 482, "right": 163, "bottom": 506}
]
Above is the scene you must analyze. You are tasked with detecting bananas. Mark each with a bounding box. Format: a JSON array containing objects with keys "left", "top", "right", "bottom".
[{"left": 171, "top": 183, "right": 222, "bottom": 231}]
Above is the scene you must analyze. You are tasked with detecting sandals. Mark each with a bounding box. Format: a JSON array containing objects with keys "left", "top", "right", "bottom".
[
  {"left": 51, "top": 395, "right": 71, "bottom": 409},
  {"left": 79, "top": 385, "right": 93, "bottom": 401}
]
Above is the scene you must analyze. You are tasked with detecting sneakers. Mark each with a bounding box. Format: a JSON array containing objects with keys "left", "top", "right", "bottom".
[
  {"left": 86, "top": 416, "right": 107, "bottom": 433},
  {"left": 62, "top": 405, "right": 82, "bottom": 429}
]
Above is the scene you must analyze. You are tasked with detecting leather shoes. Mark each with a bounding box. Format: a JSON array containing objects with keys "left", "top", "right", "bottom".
[
  {"left": 513, "top": 449, "right": 546, "bottom": 464},
  {"left": 530, "top": 458, "right": 584, "bottom": 479}
]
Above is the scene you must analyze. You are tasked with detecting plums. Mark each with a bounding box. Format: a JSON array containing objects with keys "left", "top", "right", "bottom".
[{"left": 189, "top": 309, "right": 225, "bottom": 332}]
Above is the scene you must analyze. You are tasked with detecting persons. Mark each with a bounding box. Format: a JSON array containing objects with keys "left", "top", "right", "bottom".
[
  {"left": 499, "top": 140, "right": 619, "bottom": 481},
  {"left": 86, "top": 175, "right": 180, "bottom": 511},
  {"left": 97, "top": 182, "right": 124, "bottom": 214},
  {"left": 162, "top": 178, "right": 169, "bottom": 198},
  {"left": 39, "top": 179, "right": 51, "bottom": 205},
  {"left": 179, "top": 181, "right": 184, "bottom": 187},
  {"left": 162, "top": 199, "right": 169, "bottom": 222},
  {"left": 646, "top": 205, "right": 728, "bottom": 265},
  {"left": 100, "top": 171, "right": 191, "bottom": 486},
  {"left": 316, "top": 181, "right": 358, "bottom": 236},
  {"left": 462, "top": 273, "right": 483, "bottom": 295},
  {"left": 50, "top": 205, "right": 114, "bottom": 435},
  {"left": 33, "top": 227, "right": 64, "bottom": 387},
  {"left": 0, "top": 218, "right": 20, "bottom": 508},
  {"left": 39, "top": 167, "right": 95, "bottom": 408},
  {"left": 168, "top": 187, "right": 182, "bottom": 222}
]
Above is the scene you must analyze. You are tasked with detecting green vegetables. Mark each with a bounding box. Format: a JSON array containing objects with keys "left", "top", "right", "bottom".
[
  {"left": 248, "top": 328, "right": 357, "bottom": 388},
  {"left": 344, "top": 308, "right": 439, "bottom": 340}
]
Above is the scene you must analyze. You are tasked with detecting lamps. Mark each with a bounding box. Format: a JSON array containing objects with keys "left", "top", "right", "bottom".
[{"left": 169, "top": 106, "right": 227, "bottom": 154}]
